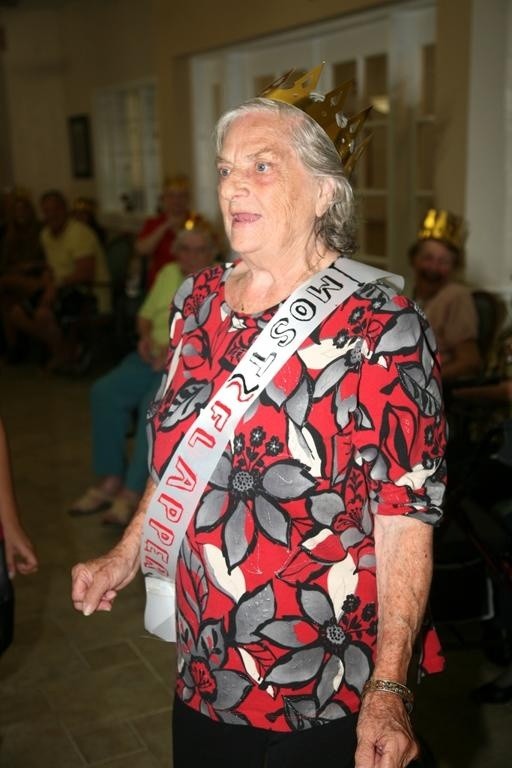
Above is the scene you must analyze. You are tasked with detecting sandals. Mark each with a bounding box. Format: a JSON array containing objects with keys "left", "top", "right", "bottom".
[{"left": 62, "top": 478, "right": 136, "bottom": 527}]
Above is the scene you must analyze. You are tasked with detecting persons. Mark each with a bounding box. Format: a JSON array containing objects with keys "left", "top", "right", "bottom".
[{"left": 71, "top": 60, "right": 450, "bottom": 768}]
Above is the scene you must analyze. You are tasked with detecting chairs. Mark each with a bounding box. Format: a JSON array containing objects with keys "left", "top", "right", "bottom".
[
  {"left": 429, "top": 290, "right": 512, "bottom": 392},
  {"left": 56, "top": 233, "right": 141, "bottom": 379}
]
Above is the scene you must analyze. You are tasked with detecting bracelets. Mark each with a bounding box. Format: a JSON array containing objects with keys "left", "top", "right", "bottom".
[{"left": 360, "top": 680, "right": 416, "bottom": 714}]
